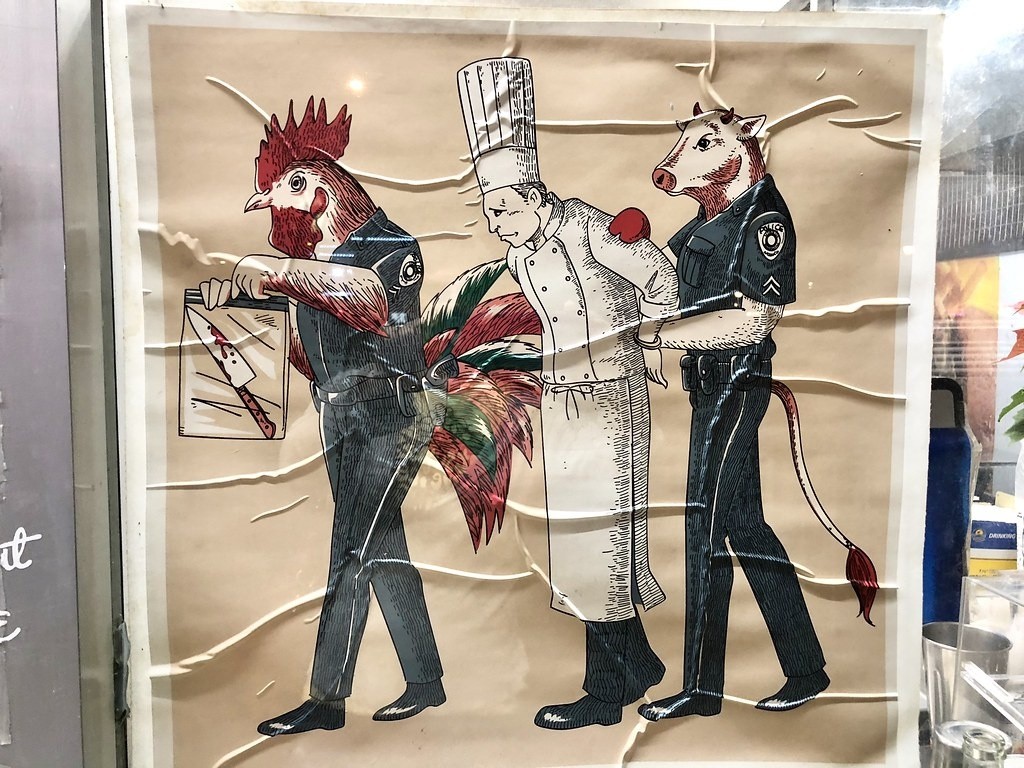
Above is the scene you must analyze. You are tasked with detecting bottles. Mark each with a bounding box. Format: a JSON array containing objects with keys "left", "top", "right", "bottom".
[{"left": 960, "top": 727, "right": 1008, "bottom": 768}]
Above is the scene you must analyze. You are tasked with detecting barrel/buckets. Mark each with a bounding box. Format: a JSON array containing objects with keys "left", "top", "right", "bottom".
[{"left": 919, "top": 621, "right": 1013, "bottom": 729}]
